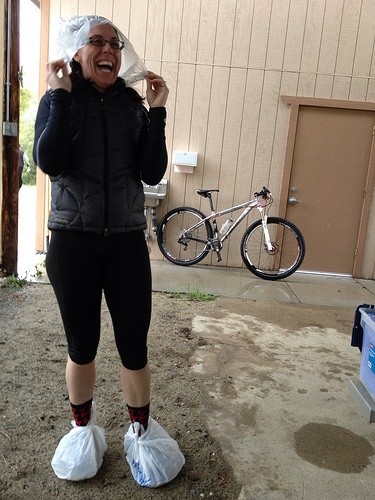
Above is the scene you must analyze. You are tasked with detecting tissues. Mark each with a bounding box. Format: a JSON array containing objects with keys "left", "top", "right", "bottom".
[{"left": 172, "top": 150, "right": 199, "bottom": 174}]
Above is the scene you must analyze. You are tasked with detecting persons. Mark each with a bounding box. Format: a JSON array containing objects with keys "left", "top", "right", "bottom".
[
  {"left": 19, "top": 144, "right": 30, "bottom": 188},
  {"left": 33, "top": 14, "right": 187, "bottom": 489}
]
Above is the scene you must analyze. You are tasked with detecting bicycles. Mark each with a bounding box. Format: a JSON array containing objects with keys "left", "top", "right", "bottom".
[{"left": 156, "top": 183, "right": 306, "bottom": 281}]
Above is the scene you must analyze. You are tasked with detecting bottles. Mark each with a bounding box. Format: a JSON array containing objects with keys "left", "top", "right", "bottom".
[{"left": 219, "top": 218, "right": 234, "bottom": 235}]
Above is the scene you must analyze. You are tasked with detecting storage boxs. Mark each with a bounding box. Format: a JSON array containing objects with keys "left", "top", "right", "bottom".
[{"left": 355, "top": 301, "right": 375, "bottom": 400}]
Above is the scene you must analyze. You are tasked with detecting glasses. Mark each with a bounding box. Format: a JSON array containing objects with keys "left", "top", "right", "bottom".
[{"left": 84, "top": 37, "right": 125, "bottom": 50}]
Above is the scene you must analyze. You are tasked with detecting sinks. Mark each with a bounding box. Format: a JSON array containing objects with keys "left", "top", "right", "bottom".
[{"left": 141, "top": 179, "right": 168, "bottom": 199}]
